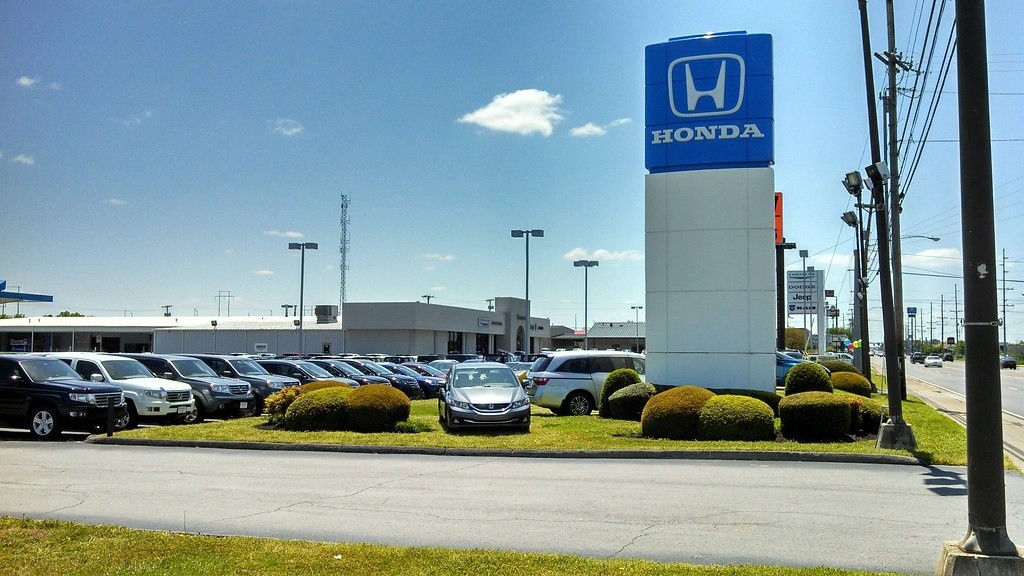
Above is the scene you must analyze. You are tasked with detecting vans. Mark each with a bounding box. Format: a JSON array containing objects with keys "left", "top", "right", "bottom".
[{"left": 525, "top": 350, "right": 644, "bottom": 417}]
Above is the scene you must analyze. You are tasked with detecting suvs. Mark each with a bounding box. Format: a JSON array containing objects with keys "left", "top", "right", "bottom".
[
  {"left": 0, "top": 353, "right": 127, "bottom": 442},
  {"left": 171, "top": 353, "right": 301, "bottom": 418},
  {"left": 96, "top": 353, "right": 256, "bottom": 421},
  {"left": 19, "top": 353, "right": 197, "bottom": 432}
]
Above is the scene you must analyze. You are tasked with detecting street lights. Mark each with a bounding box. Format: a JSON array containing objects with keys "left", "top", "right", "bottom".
[
  {"left": 807, "top": 264, "right": 815, "bottom": 349},
  {"left": 863, "top": 235, "right": 941, "bottom": 383},
  {"left": 799, "top": 249, "right": 809, "bottom": 351},
  {"left": 574, "top": 260, "right": 600, "bottom": 350},
  {"left": 630, "top": 306, "right": 644, "bottom": 353},
  {"left": 290, "top": 243, "right": 318, "bottom": 360},
  {"left": 511, "top": 229, "right": 545, "bottom": 363}
]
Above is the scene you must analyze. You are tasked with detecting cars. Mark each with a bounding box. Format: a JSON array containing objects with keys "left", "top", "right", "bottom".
[
  {"left": 869, "top": 347, "right": 885, "bottom": 358},
  {"left": 1000, "top": 357, "right": 1016, "bottom": 370},
  {"left": 231, "top": 347, "right": 646, "bottom": 400},
  {"left": 776, "top": 348, "right": 855, "bottom": 387},
  {"left": 438, "top": 362, "right": 531, "bottom": 433},
  {"left": 923, "top": 355, "right": 943, "bottom": 368},
  {"left": 910, "top": 351, "right": 924, "bottom": 364},
  {"left": 943, "top": 352, "right": 954, "bottom": 362}
]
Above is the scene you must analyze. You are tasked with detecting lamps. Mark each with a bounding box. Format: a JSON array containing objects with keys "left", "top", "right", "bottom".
[
  {"left": 293, "top": 320, "right": 300, "bottom": 326},
  {"left": 210, "top": 320, "right": 217, "bottom": 326}
]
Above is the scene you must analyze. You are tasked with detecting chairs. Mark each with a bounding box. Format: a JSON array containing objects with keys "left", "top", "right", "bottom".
[
  {"left": 486, "top": 372, "right": 506, "bottom": 382},
  {"left": 456, "top": 373, "right": 469, "bottom": 387},
  {"left": 472, "top": 373, "right": 481, "bottom": 383}
]
